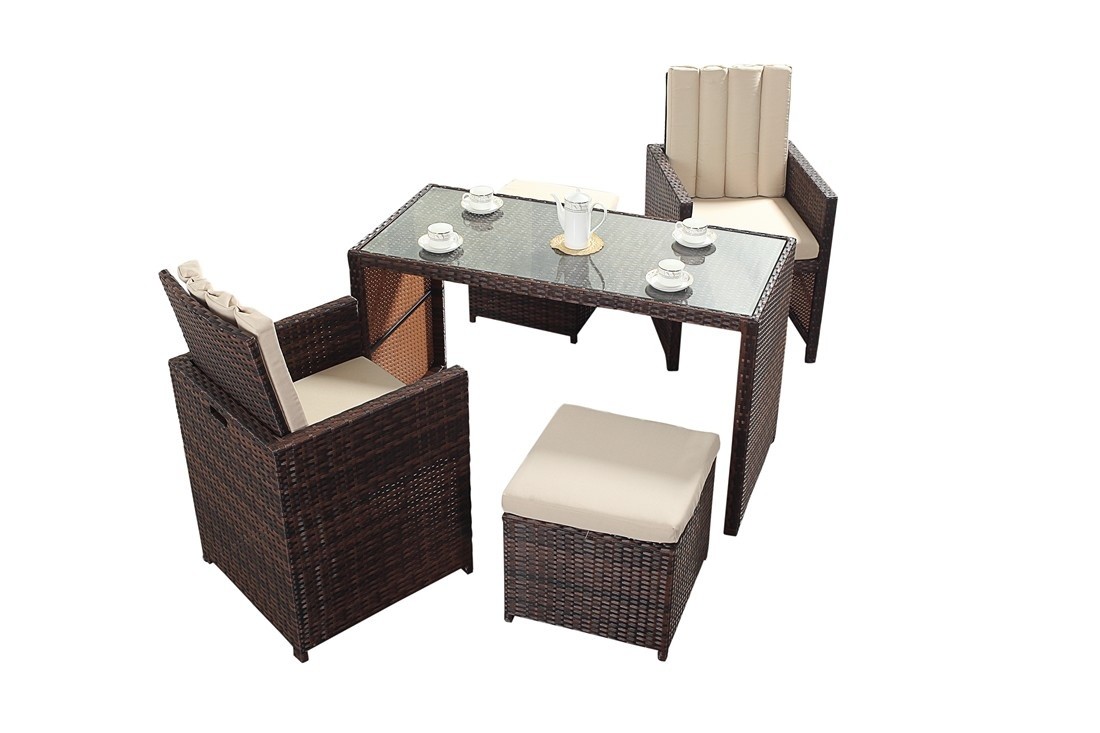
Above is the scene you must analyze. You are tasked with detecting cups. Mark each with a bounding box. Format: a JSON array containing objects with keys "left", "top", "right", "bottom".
[
  {"left": 427, "top": 222, "right": 458, "bottom": 247},
  {"left": 462, "top": 186, "right": 495, "bottom": 211},
  {"left": 675, "top": 218, "right": 708, "bottom": 244},
  {"left": 657, "top": 258, "right": 685, "bottom": 284}
]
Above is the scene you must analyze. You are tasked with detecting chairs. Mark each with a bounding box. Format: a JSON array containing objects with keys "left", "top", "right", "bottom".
[
  {"left": 643, "top": 64, "right": 839, "bottom": 371},
  {"left": 158, "top": 262, "right": 475, "bottom": 662}
]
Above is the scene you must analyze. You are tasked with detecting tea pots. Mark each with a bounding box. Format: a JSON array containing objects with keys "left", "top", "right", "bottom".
[{"left": 550, "top": 188, "right": 607, "bottom": 249}]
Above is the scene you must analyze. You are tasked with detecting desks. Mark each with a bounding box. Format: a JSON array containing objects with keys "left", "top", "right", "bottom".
[{"left": 347, "top": 183, "right": 798, "bottom": 538}]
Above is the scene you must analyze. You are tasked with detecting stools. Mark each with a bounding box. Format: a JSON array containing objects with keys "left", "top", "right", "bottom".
[{"left": 501, "top": 403, "right": 721, "bottom": 662}]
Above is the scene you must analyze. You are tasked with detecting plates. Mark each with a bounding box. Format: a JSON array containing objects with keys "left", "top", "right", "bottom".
[
  {"left": 672, "top": 228, "right": 717, "bottom": 248},
  {"left": 461, "top": 197, "right": 504, "bottom": 215},
  {"left": 646, "top": 268, "right": 694, "bottom": 293},
  {"left": 418, "top": 233, "right": 463, "bottom": 253}
]
